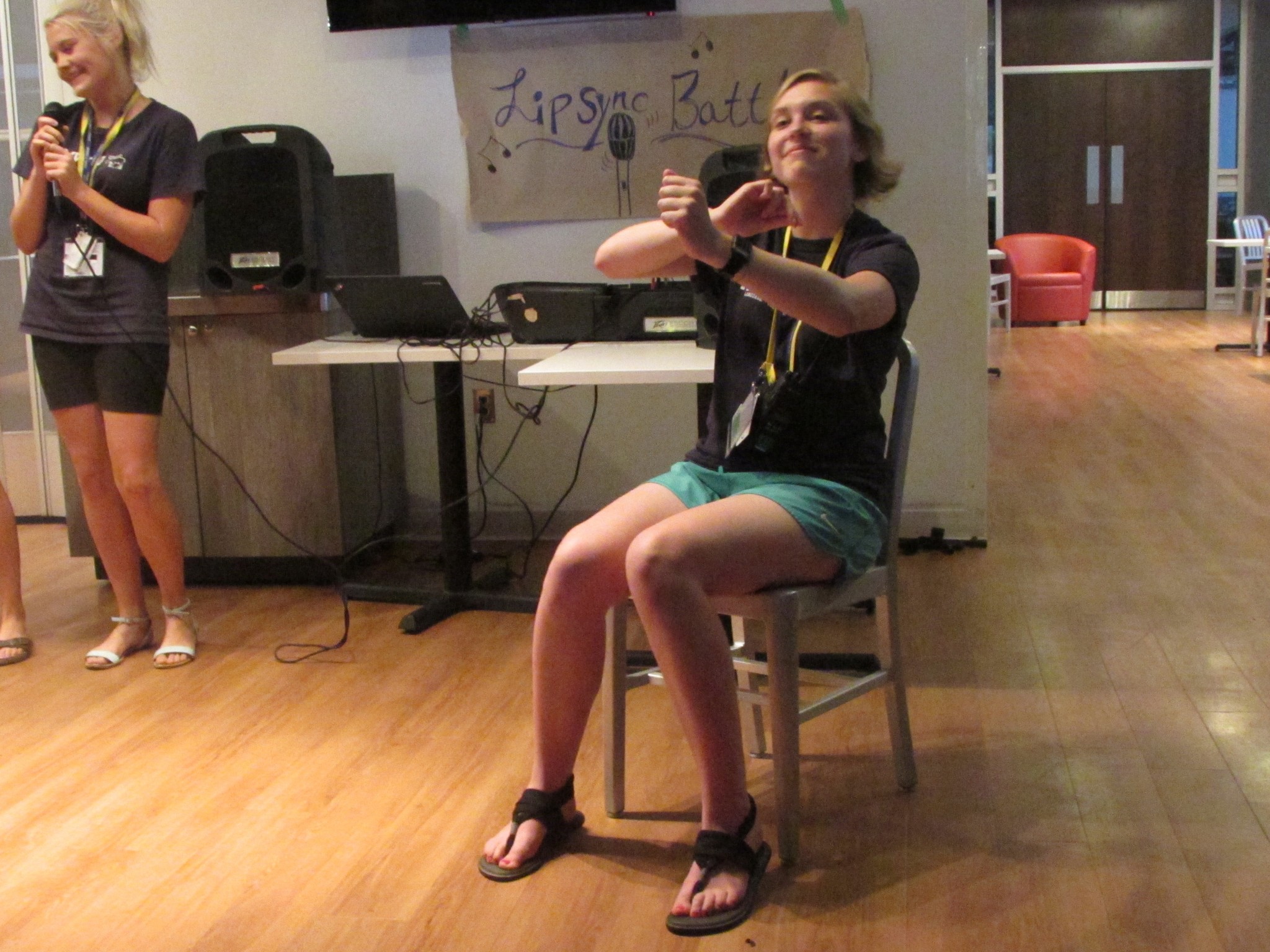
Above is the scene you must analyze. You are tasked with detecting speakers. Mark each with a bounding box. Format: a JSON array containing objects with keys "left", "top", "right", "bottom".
[{"left": 193, "top": 124, "right": 342, "bottom": 297}]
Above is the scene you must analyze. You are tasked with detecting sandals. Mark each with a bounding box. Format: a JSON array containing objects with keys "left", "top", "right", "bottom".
[
  {"left": 152, "top": 599, "right": 196, "bottom": 668},
  {"left": 666, "top": 793, "right": 771, "bottom": 936},
  {"left": 478, "top": 777, "right": 586, "bottom": 881},
  {"left": 84, "top": 615, "right": 149, "bottom": 671}
]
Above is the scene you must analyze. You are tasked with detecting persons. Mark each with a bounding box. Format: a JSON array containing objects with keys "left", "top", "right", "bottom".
[
  {"left": 11, "top": 1, "right": 208, "bottom": 669},
  {"left": 478, "top": 69, "right": 920, "bottom": 936},
  {"left": 0, "top": 484, "right": 31, "bottom": 668}
]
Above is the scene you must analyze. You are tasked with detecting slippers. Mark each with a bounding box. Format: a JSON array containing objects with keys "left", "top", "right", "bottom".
[{"left": 0, "top": 637, "right": 33, "bottom": 667}]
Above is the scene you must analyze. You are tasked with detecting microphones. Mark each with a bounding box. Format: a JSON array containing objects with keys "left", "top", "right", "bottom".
[{"left": 44, "top": 102, "right": 65, "bottom": 211}]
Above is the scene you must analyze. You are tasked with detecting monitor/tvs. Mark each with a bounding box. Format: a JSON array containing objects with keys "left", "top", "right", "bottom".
[{"left": 325, "top": 0, "right": 676, "bottom": 34}]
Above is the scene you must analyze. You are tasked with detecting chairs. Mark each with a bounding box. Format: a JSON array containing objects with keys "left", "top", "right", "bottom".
[
  {"left": 987, "top": 249, "right": 1011, "bottom": 332},
  {"left": 994, "top": 233, "right": 1095, "bottom": 327},
  {"left": 601, "top": 338, "right": 919, "bottom": 867},
  {"left": 1234, "top": 216, "right": 1270, "bottom": 356}
]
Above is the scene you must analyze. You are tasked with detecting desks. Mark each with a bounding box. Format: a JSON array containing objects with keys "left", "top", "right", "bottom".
[
  {"left": 271, "top": 328, "right": 881, "bottom": 674},
  {"left": 1206, "top": 238, "right": 1270, "bottom": 352}
]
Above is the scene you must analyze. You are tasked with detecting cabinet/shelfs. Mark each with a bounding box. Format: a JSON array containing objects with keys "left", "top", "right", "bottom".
[{"left": 59, "top": 275, "right": 405, "bottom": 586}]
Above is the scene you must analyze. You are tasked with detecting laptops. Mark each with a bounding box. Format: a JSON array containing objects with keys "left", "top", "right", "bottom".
[{"left": 326, "top": 275, "right": 510, "bottom": 338}]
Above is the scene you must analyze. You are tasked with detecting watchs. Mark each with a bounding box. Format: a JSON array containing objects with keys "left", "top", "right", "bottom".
[{"left": 717, "top": 235, "right": 753, "bottom": 278}]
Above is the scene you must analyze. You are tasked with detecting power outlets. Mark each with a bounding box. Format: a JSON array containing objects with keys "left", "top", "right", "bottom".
[{"left": 473, "top": 388, "right": 495, "bottom": 423}]
